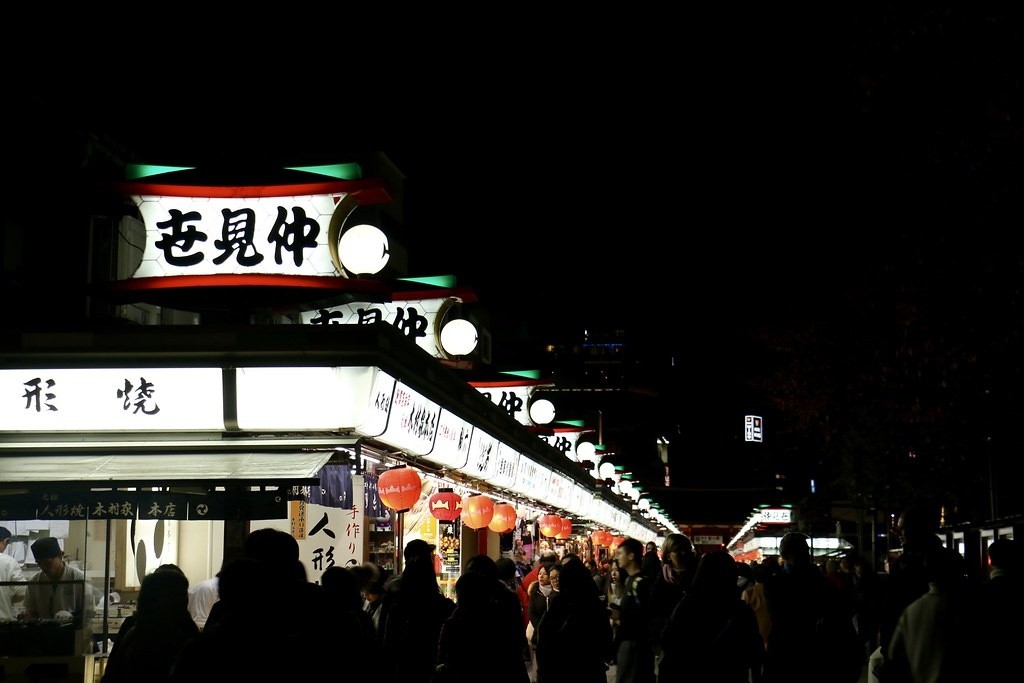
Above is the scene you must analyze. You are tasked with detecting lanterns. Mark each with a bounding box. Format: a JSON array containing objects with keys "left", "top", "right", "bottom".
[
  {"left": 602, "top": 531, "right": 613, "bottom": 549},
  {"left": 487, "top": 501, "right": 517, "bottom": 534},
  {"left": 554, "top": 516, "right": 572, "bottom": 541},
  {"left": 591, "top": 529, "right": 607, "bottom": 547},
  {"left": 461, "top": 493, "right": 494, "bottom": 531},
  {"left": 429, "top": 488, "right": 463, "bottom": 524},
  {"left": 541, "top": 512, "right": 562, "bottom": 539},
  {"left": 614, "top": 535, "right": 625, "bottom": 547},
  {"left": 378, "top": 464, "right": 422, "bottom": 513}
]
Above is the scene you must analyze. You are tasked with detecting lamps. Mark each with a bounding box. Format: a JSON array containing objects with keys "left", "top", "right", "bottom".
[
  {"left": 441, "top": 319, "right": 478, "bottom": 373},
  {"left": 338, "top": 223, "right": 392, "bottom": 301},
  {"left": 599, "top": 462, "right": 615, "bottom": 490},
  {"left": 619, "top": 480, "right": 681, "bottom": 534},
  {"left": 530, "top": 399, "right": 556, "bottom": 442},
  {"left": 577, "top": 442, "right": 595, "bottom": 471}
]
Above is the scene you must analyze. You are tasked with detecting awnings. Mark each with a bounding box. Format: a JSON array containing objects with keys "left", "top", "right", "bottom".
[{"left": 0, "top": 451, "right": 338, "bottom": 486}]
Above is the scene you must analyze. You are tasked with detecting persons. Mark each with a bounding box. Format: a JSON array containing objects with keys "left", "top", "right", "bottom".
[
  {"left": 100, "top": 528, "right": 1024, "bottom": 683},
  {"left": 0, "top": 526, "right": 27, "bottom": 623},
  {"left": 16, "top": 537, "right": 97, "bottom": 620}
]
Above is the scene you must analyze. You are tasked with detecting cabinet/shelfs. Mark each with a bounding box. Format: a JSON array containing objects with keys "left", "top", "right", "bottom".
[{"left": 362, "top": 475, "right": 397, "bottom": 579}]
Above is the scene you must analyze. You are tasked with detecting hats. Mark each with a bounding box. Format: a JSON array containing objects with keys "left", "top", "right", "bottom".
[
  {"left": 404, "top": 540, "right": 436, "bottom": 560},
  {"left": 31, "top": 538, "right": 60, "bottom": 558}
]
